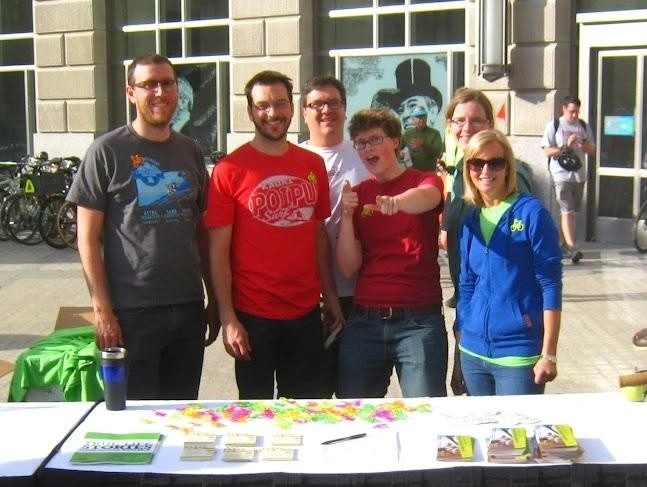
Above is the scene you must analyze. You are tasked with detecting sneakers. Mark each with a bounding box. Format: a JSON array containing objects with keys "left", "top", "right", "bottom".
[
  {"left": 444, "top": 292, "right": 457, "bottom": 308},
  {"left": 569, "top": 248, "right": 584, "bottom": 264}
]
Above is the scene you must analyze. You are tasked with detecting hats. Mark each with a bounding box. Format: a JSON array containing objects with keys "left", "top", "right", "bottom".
[
  {"left": 410, "top": 106, "right": 428, "bottom": 118},
  {"left": 389, "top": 56, "right": 445, "bottom": 112}
]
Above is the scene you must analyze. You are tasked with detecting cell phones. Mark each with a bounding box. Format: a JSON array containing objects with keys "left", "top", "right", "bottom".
[{"left": 577, "top": 136, "right": 588, "bottom": 143}]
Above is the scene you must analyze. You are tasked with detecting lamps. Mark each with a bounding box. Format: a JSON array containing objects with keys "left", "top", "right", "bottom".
[{"left": 476, "top": 0, "right": 511, "bottom": 85}]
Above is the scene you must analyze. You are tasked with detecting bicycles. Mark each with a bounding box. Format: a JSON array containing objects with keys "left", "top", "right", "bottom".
[
  {"left": 0, "top": 149, "right": 82, "bottom": 253},
  {"left": 631, "top": 199, "right": 647, "bottom": 255}
]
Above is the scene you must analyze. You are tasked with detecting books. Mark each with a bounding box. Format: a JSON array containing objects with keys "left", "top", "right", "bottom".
[
  {"left": 70, "top": 431, "right": 164, "bottom": 464},
  {"left": 435, "top": 421, "right": 587, "bottom": 464}
]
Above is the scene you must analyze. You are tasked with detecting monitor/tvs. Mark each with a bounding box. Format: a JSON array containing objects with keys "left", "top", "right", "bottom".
[{"left": 603, "top": 114, "right": 634, "bottom": 136}]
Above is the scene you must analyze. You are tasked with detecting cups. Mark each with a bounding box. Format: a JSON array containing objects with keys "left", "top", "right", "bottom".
[{"left": 100, "top": 346, "right": 128, "bottom": 410}]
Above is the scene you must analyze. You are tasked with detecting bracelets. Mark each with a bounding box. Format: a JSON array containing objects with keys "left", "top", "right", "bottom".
[{"left": 540, "top": 351, "right": 558, "bottom": 364}]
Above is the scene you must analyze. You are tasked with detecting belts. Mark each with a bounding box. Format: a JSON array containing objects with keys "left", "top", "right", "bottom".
[{"left": 348, "top": 300, "right": 442, "bottom": 323}]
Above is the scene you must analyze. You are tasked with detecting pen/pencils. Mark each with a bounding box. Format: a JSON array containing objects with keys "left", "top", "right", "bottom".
[{"left": 322, "top": 434, "right": 365, "bottom": 445}]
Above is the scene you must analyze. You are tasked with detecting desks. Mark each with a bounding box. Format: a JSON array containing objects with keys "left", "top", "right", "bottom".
[
  {"left": 1, "top": 400, "right": 98, "bottom": 486},
  {"left": 37, "top": 392, "right": 647, "bottom": 486}
]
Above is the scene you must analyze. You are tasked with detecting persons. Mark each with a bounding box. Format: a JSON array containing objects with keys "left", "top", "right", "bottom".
[
  {"left": 539, "top": 95, "right": 596, "bottom": 262},
  {"left": 64, "top": 52, "right": 221, "bottom": 401},
  {"left": 334, "top": 110, "right": 449, "bottom": 399},
  {"left": 444, "top": 87, "right": 531, "bottom": 311},
  {"left": 201, "top": 69, "right": 343, "bottom": 400},
  {"left": 395, "top": 108, "right": 445, "bottom": 176},
  {"left": 448, "top": 132, "right": 563, "bottom": 396},
  {"left": 294, "top": 76, "right": 374, "bottom": 398}
]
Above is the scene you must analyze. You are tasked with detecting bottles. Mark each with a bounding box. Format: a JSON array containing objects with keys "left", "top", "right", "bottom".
[
  {"left": 401, "top": 146, "right": 412, "bottom": 167},
  {"left": 435, "top": 157, "right": 449, "bottom": 177}
]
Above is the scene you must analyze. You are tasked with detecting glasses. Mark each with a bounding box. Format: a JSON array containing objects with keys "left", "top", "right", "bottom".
[
  {"left": 464, "top": 155, "right": 507, "bottom": 171},
  {"left": 249, "top": 99, "right": 291, "bottom": 110},
  {"left": 302, "top": 97, "right": 344, "bottom": 111},
  {"left": 130, "top": 78, "right": 179, "bottom": 92},
  {"left": 351, "top": 133, "right": 392, "bottom": 150},
  {"left": 448, "top": 114, "right": 491, "bottom": 129}
]
